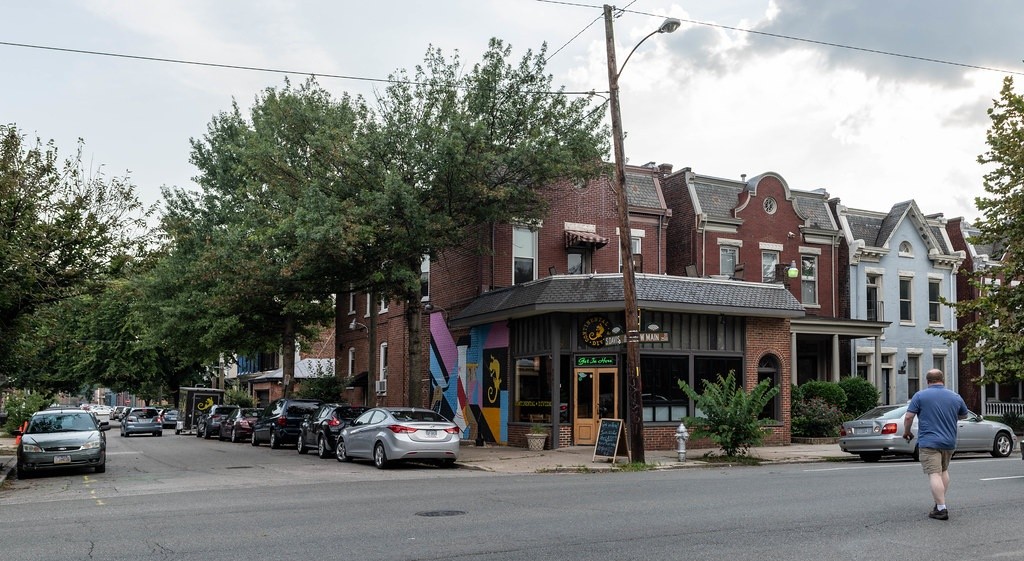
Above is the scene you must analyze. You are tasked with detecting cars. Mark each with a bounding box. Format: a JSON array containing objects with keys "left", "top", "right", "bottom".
[
  {"left": 838, "top": 399, "right": 1018, "bottom": 463},
  {"left": 297, "top": 403, "right": 373, "bottom": 458},
  {"left": 336, "top": 405, "right": 460, "bottom": 469},
  {"left": 13, "top": 408, "right": 112, "bottom": 480},
  {"left": 120, "top": 407, "right": 164, "bottom": 437},
  {"left": 219, "top": 406, "right": 264, "bottom": 443},
  {"left": 80, "top": 402, "right": 179, "bottom": 429}
]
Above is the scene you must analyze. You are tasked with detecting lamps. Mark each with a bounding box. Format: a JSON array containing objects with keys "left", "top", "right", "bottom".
[
  {"left": 785, "top": 260, "right": 800, "bottom": 278},
  {"left": 349, "top": 318, "right": 369, "bottom": 334},
  {"left": 898, "top": 359, "right": 907, "bottom": 374},
  {"left": 720, "top": 316, "right": 725, "bottom": 324},
  {"left": 424, "top": 302, "right": 449, "bottom": 320}
]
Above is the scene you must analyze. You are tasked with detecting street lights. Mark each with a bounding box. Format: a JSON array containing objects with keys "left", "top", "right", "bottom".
[{"left": 610, "top": 16, "right": 683, "bottom": 465}]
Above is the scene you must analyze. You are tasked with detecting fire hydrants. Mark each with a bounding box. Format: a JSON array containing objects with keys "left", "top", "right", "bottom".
[{"left": 674, "top": 422, "right": 690, "bottom": 462}]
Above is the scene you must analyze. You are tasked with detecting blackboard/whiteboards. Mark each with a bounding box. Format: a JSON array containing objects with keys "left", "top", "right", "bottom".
[{"left": 593, "top": 418, "right": 630, "bottom": 458}]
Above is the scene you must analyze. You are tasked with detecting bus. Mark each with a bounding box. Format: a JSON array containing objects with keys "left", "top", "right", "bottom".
[{"left": 196, "top": 402, "right": 242, "bottom": 438}]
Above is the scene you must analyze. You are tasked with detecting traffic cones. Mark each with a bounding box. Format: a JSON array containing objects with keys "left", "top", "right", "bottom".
[
  {"left": 23, "top": 421, "right": 28, "bottom": 432},
  {"left": 16, "top": 426, "right": 22, "bottom": 445}
]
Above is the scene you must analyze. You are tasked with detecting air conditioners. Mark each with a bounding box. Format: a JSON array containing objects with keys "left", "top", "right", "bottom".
[{"left": 376, "top": 380, "right": 386, "bottom": 392}]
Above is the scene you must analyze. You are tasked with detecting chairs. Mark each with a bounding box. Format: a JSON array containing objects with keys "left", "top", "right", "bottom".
[
  {"left": 71, "top": 418, "right": 87, "bottom": 427},
  {"left": 529, "top": 414, "right": 543, "bottom": 423}
]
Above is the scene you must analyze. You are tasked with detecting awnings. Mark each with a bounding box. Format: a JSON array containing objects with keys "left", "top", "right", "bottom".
[{"left": 345, "top": 372, "right": 368, "bottom": 387}]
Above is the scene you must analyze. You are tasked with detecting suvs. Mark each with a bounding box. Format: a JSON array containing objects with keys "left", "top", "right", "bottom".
[{"left": 250, "top": 397, "right": 326, "bottom": 449}]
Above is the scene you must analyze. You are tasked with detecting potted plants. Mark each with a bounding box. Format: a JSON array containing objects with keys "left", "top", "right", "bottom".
[{"left": 524, "top": 422, "right": 548, "bottom": 452}]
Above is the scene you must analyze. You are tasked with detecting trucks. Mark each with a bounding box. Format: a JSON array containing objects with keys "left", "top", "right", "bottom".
[{"left": 175, "top": 384, "right": 226, "bottom": 434}]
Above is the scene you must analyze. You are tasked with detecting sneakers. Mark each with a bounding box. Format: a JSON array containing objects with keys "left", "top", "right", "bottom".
[{"left": 928, "top": 503, "right": 949, "bottom": 520}]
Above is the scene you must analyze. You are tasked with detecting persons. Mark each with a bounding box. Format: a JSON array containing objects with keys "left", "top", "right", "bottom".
[{"left": 903, "top": 368, "right": 969, "bottom": 520}]
